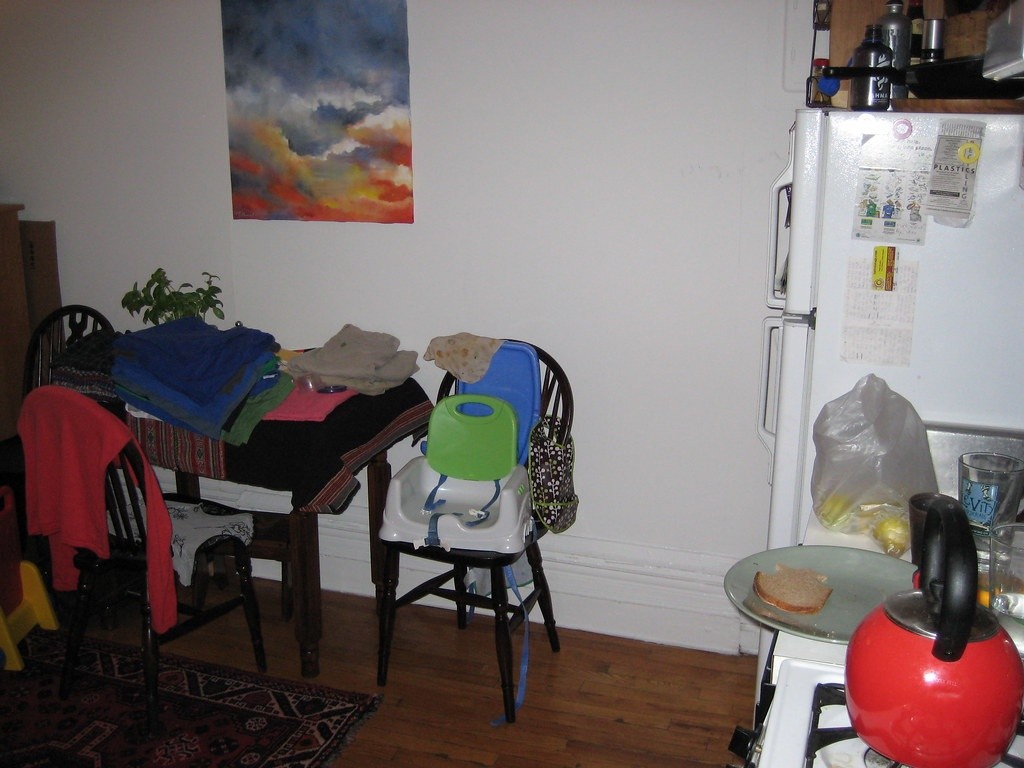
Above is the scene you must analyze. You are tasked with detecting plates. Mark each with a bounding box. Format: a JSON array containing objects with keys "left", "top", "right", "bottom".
[{"left": 724, "top": 545, "right": 919, "bottom": 645}]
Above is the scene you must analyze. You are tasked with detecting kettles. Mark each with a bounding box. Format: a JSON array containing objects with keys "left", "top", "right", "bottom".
[{"left": 845, "top": 492, "right": 1024, "bottom": 768}]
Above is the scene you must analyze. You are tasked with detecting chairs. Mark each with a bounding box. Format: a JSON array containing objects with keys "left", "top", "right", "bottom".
[
  {"left": 16, "top": 385, "right": 267, "bottom": 729},
  {"left": 376, "top": 339, "right": 574, "bottom": 725},
  {"left": 27, "top": 304, "right": 114, "bottom": 391},
  {"left": 380, "top": 342, "right": 533, "bottom": 554}
]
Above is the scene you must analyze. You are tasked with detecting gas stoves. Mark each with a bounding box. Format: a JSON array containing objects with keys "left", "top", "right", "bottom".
[{"left": 758, "top": 660, "right": 1024, "bottom": 768}]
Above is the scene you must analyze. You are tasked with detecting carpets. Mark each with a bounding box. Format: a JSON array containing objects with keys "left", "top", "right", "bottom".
[{"left": 0, "top": 624, "right": 382, "bottom": 768}]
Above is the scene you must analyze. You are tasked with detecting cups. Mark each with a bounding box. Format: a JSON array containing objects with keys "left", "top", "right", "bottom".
[
  {"left": 909, "top": 494, "right": 955, "bottom": 567},
  {"left": 957, "top": 452, "right": 1024, "bottom": 538},
  {"left": 988, "top": 524, "right": 1024, "bottom": 619}
]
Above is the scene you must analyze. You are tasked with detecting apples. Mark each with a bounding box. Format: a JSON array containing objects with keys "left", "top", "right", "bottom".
[{"left": 818, "top": 493, "right": 910, "bottom": 557}]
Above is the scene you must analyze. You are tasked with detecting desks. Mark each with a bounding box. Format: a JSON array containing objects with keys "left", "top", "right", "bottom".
[
  {"left": 86, "top": 347, "right": 417, "bottom": 681},
  {"left": 724, "top": 546, "right": 919, "bottom": 665}
]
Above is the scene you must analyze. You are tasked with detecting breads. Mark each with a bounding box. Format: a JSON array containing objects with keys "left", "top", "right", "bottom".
[{"left": 753, "top": 567, "right": 834, "bottom": 615}]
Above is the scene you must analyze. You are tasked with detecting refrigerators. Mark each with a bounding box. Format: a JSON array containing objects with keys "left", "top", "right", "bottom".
[{"left": 754, "top": 107, "right": 1024, "bottom": 551}]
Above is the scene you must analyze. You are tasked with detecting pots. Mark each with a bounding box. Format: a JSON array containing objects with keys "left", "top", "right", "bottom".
[{"left": 822, "top": 56, "right": 1024, "bottom": 100}]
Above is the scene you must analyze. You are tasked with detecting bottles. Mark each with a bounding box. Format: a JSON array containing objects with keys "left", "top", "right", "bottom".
[
  {"left": 812, "top": 59, "right": 831, "bottom": 105},
  {"left": 877, "top": 0, "right": 912, "bottom": 98},
  {"left": 849, "top": 23, "right": 893, "bottom": 111}
]
